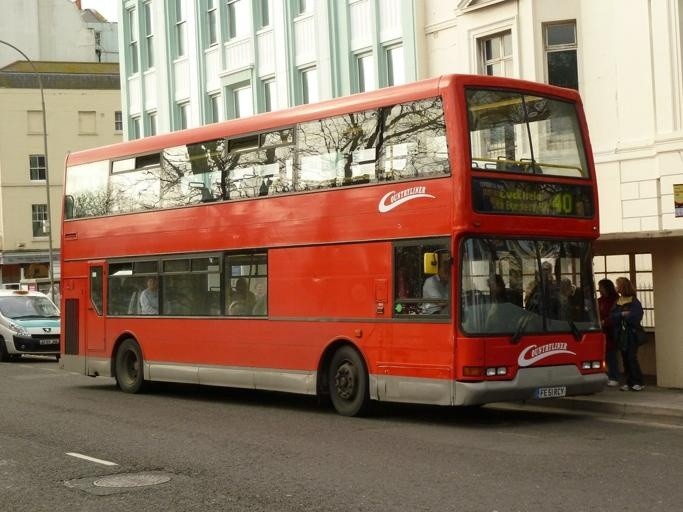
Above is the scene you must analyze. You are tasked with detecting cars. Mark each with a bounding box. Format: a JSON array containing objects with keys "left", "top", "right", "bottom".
[{"left": 0, "top": 289, "right": 60, "bottom": 361}]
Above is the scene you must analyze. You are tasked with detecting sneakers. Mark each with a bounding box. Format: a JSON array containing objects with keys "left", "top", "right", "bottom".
[
  {"left": 607, "top": 380, "right": 618, "bottom": 386},
  {"left": 620, "top": 384, "right": 646, "bottom": 391}
]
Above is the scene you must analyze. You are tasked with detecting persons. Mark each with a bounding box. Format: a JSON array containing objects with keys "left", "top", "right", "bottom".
[
  {"left": 597, "top": 279, "right": 619, "bottom": 387},
  {"left": 485, "top": 274, "right": 507, "bottom": 302},
  {"left": 420, "top": 252, "right": 451, "bottom": 316},
  {"left": 138, "top": 276, "right": 171, "bottom": 315},
  {"left": 527, "top": 262, "right": 583, "bottom": 319},
  {"left": 609, "top": 276, "right": 646, "bottom": 391},
  {"left": 232, "top": 277, "right": 256, "bottom": 305},
  {"left": 254, "top": 280, "right": 264, "bottom": 298}
]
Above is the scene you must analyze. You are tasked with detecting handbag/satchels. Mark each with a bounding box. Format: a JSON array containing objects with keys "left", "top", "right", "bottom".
[{"left": 618, "top": 322, "right": 648, "bottom": 350}]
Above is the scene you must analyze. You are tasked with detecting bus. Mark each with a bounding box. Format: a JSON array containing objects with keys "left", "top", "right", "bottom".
[{"left": 60, "top": 74, "right": 609, "bottom": 415}]
